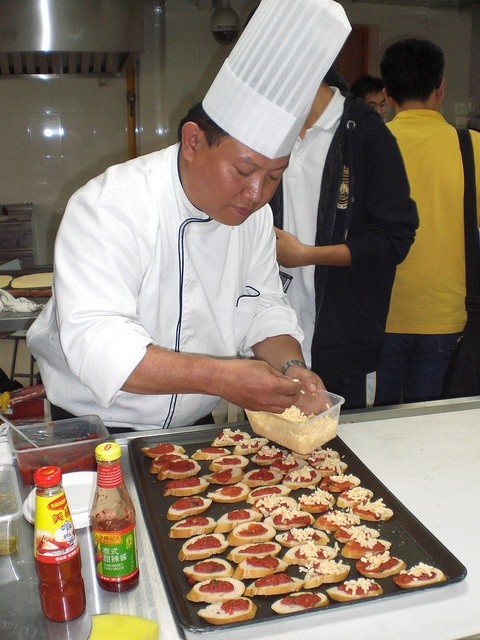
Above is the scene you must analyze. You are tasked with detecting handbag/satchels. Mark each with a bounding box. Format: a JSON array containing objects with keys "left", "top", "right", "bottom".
[{"left": 447, "top": 325, "right": 480, "bottom": 398}]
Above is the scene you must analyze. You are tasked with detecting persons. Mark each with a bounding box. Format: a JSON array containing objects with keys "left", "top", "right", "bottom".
[
  {"left": 272, "top": 78, "right": 420, "bottom": 412},
  {"left": 375, "top": 39, "right": 480, "bottom": 405},
  {"left": 24, "top": 1, "right": 333, "bottom": 445},
  {"left": 349, "top": 74, "right": 390, "bottom": 122}
]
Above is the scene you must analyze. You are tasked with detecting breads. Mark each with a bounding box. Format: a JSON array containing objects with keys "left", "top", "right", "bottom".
[{"left": 143, "top": 425, "right": 448, "bottom": 624}]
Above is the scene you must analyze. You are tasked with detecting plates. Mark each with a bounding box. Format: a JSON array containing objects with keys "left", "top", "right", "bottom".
[{"left": 21, "top": 469, "right": 99, "bottom": 530}]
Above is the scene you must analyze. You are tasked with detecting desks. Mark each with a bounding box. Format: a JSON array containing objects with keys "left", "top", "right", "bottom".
[{"left": 3, "top": 396, "right": 480, "bottom": 639}]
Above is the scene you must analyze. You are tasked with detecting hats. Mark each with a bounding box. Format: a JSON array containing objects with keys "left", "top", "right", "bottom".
[{"left": 203, "top": 0, "right": 353, "bottom": 160}]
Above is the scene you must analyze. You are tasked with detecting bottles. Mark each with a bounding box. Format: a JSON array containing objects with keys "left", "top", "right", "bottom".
[
  {"left": 87, "top": 441, "right": 141, "bottom": 593},
  {"left": 30, "top": 464, "right": 86, "bottom": 624}
]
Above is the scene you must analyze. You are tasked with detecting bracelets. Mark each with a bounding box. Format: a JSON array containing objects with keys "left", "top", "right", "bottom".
[{"left": 280, "top": 359, "right": 307, "bottom": 375}]
